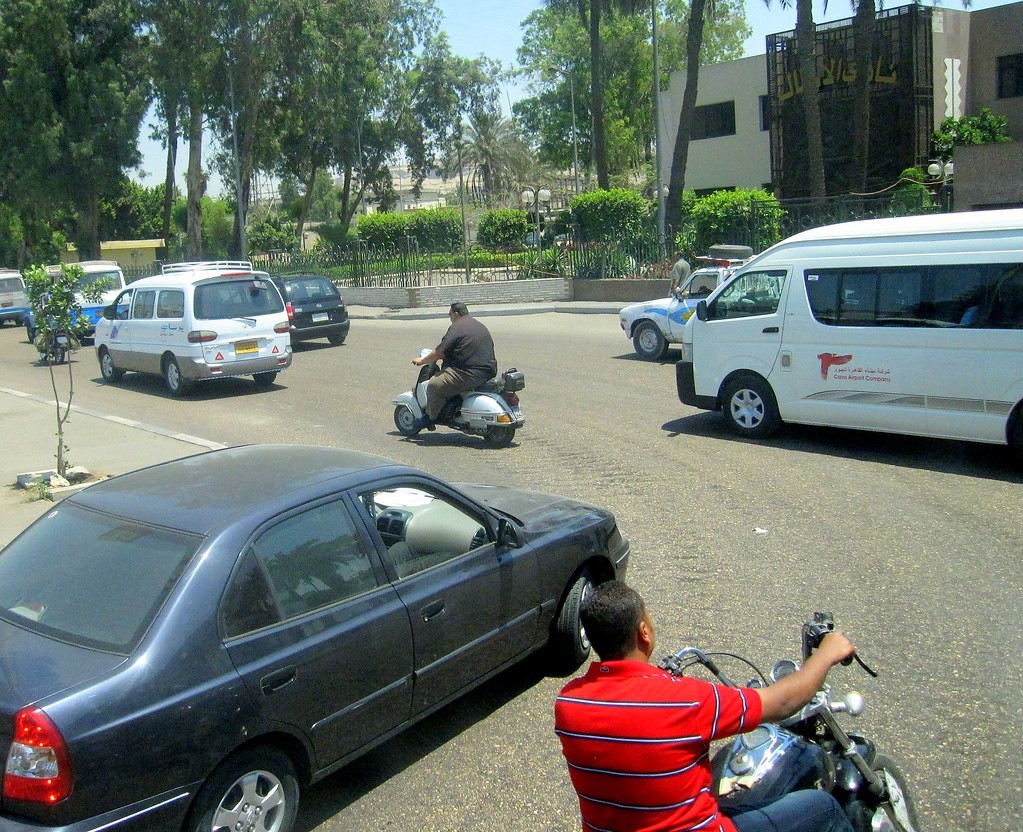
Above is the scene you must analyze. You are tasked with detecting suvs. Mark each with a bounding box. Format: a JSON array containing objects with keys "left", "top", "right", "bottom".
[{"left": 268, "top": 270, "right": 350, "bottom": 351}]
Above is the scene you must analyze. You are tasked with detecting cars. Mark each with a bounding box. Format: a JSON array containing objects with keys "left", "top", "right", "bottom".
[
  {"left": 1, "top": 441, "right": 630, "bottom": 832},
  {"left": 525, "top": 208, "right": 575, "bottom": 251}
]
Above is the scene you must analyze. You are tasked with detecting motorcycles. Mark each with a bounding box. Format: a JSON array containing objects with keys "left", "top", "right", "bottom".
[
  {"left": 655, "top": 608, "right": 922, "bottom": 832},
  {"left": 30, "top": 309, "right": 69, "bottom": 364}
]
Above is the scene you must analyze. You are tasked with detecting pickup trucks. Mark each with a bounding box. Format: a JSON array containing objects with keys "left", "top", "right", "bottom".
[{"left": 619, "top": 245, "right": 857, "bottom": 361}]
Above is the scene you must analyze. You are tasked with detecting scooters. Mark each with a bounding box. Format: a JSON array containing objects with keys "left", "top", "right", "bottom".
[{"left": 391, "top": 349, "right": 525, "bottom": 447}]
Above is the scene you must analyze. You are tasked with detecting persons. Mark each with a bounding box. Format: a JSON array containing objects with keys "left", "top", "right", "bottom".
[
  {"left": 411, "top": 302, "right": 498, "bottom": 429},
  {"left": 668, "top": 251, "right": 691, "bottom": 298},
  {"left": 554, "top": 581, "right": 856, "bottom": 832},
  {"left": 41, "top": 285, "right": 55, "bottom": 307}
]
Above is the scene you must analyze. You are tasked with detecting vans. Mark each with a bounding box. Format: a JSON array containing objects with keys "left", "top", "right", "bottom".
[
  {"left": 92, "top": 261, "right": 294, "bottom": 398},
  {"left": 675, "top": 207, "right": 1022, "bottom": 459},
  {"left": 0, "top": 268, "right": 29, "bottom": 327},
  {"left": 25, "top": 260, "right": 130, "bottom": 343}
]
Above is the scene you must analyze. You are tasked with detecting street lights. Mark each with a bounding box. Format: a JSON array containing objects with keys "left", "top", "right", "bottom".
[
  {"left": 521, "top": 183, "right": 550, "bottom": 278},
  {"left": 927, "top": 158, "right": 955, "bottom": 213}
]
